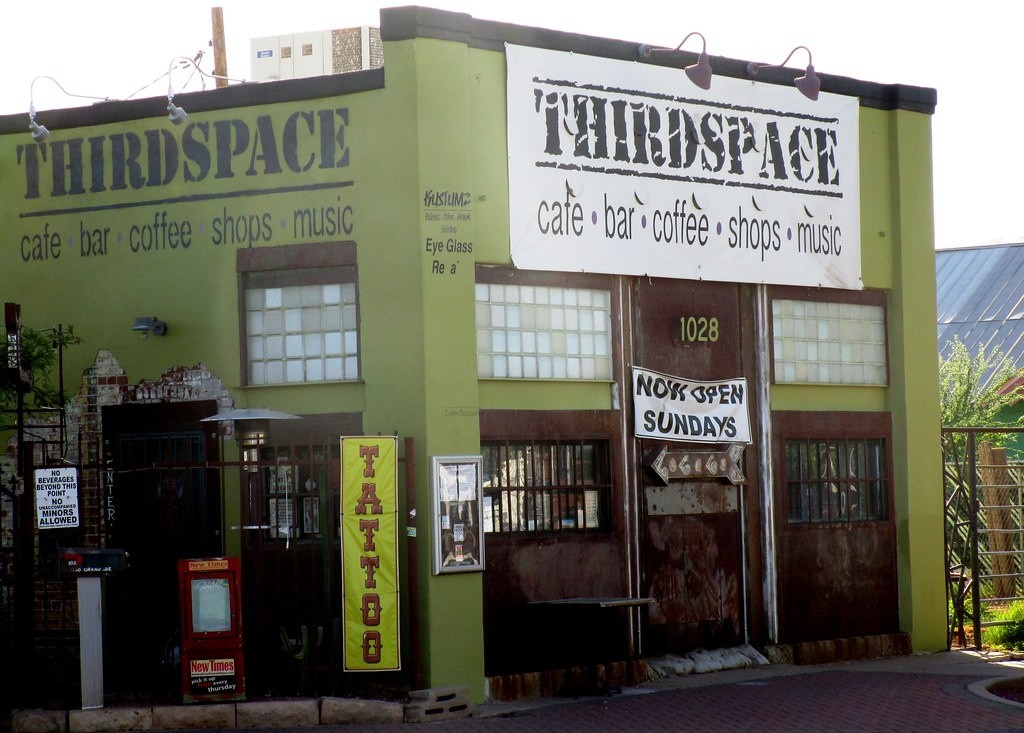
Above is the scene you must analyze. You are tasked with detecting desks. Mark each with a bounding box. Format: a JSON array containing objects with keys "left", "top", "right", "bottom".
[{"left": 526, "top": 597, "right": 653, "bottom": 697}]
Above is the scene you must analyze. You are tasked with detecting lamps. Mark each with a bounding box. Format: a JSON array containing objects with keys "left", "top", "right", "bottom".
[
  {"left": 166, "top": 57, "right": 253, "bottom": 124},
  {"left": 748, "top": 46, "right": 820, "bottom": 101},
  {"left": 200, "top": 405, "right": 305, "bottom": 449},
  {"left": 132, "top": 318, "right": 166, "bottom": 339},
  {"left": 28, "top": 77, "right": 115, "bottom": 144},
  {"left": 638, "top": 32, "right": 713, "bottom": 90}
]
